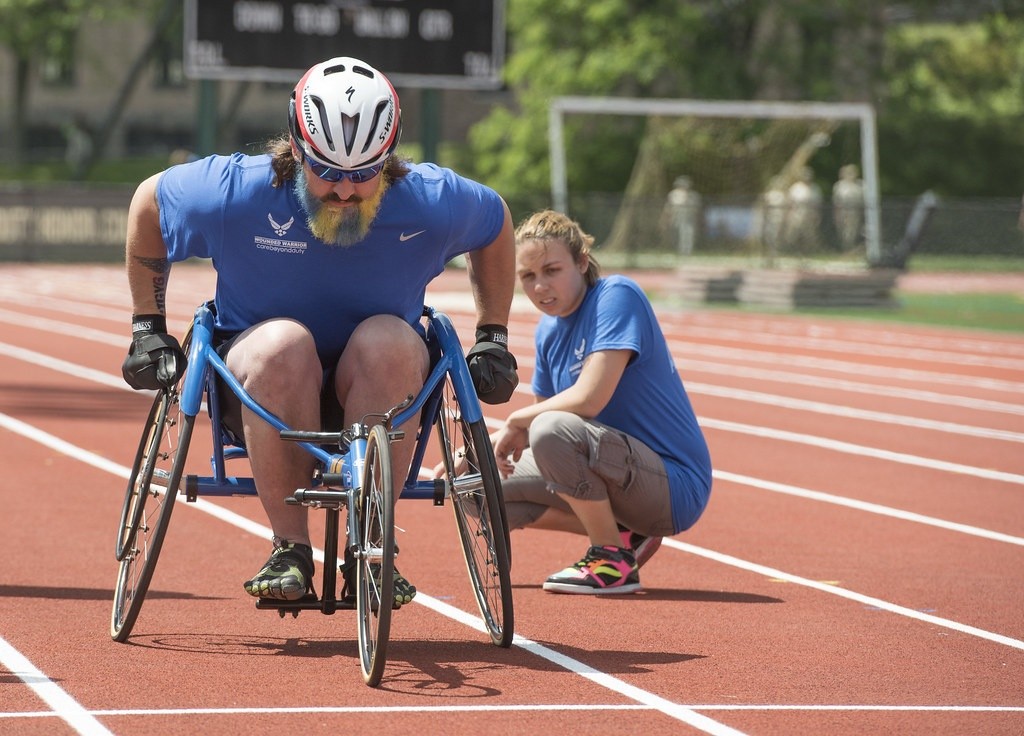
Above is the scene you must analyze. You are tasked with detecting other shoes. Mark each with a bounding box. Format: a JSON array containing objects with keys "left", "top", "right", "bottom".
[
  {"left": 243, "top": 539, "right": 313, "bottom": 601},
  {"left": 342, "top": 548, "right": 417, "bottom": 605}
]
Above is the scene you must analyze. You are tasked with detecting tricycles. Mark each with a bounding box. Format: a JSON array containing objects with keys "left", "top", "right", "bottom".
[{"left": 108, "top": 299, "right": 515, "bottom": 690}]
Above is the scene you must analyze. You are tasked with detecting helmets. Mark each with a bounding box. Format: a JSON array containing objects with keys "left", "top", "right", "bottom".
[{"left": 288, "top": 57, "right": 400, "bottom": 170}]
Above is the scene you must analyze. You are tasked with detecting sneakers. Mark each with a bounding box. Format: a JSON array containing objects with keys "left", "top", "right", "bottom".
[
  {"left": 543, "top": 534, "right": 640, "bottom": 594},
  {"left": 624, "top": 529, "right": 664, "bottom": 566}
]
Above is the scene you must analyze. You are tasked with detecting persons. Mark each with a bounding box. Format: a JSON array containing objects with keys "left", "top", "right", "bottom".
[
  {"left": 121, "top": 56, "right": 518, "bottom": 607},
  {"left": 429, "top": 211, "right": 712, "bottom": 595},
  {"left": 658, "top": 163, "right": 866, "bottom": 267}
]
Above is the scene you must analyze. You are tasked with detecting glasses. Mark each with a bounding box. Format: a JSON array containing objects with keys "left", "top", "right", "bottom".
[{"left": 295, "top": 144, "right": 385, "bottom": 182}]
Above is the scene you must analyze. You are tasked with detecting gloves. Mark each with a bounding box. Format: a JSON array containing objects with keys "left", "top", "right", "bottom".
[
  {"left": 121, "top": 314, "right": 186, "bottom": 390},
  {"left": 466, "top": 323, "right": 519, "bottom": 401}
]
